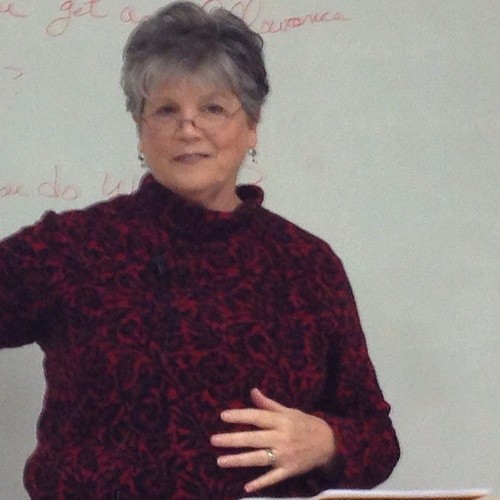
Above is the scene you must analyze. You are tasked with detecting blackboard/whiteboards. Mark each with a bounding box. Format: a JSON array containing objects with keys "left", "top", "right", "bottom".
[{"left": 0, "top": 0, "right": 494, "bottom": 499}]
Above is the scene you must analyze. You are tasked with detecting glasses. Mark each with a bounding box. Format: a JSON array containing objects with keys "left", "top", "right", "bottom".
[{"left": 139, "top": 106, "right": 241, "bottom": 130}]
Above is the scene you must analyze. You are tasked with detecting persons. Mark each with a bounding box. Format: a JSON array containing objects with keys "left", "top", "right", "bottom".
[{"left": 1, "top": 0, "right": 403, "bottom": 500}]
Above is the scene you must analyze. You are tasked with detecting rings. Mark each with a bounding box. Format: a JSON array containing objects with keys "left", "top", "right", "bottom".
[{"left": 264, "top": 446, "right": 275, "bottom": 468}]
